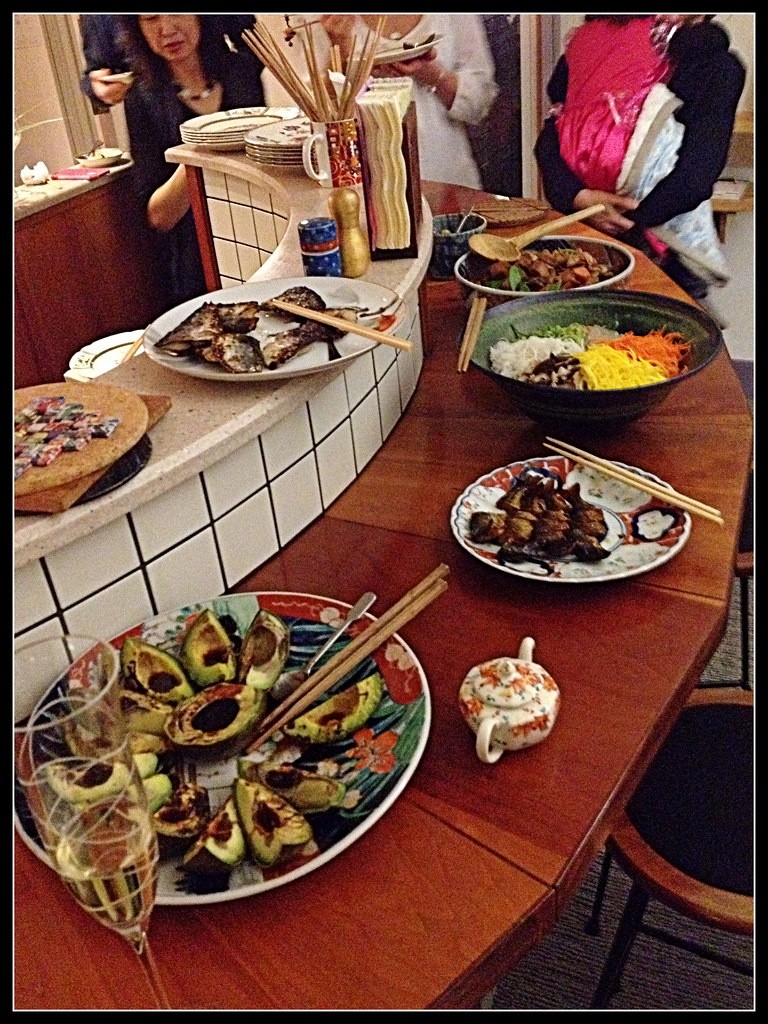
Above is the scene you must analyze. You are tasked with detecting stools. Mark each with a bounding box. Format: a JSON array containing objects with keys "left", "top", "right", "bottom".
[
  {"left": 710, "top": 177, "right": 755, "bottom": 245},
  {"left": 589, "top": 679, "right": 755, "bottom": 1011}
]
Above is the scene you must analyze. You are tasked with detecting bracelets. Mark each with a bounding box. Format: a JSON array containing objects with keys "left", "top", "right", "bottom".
[{"left": 426, "top": 71, "right": 446, "bottom": 94}]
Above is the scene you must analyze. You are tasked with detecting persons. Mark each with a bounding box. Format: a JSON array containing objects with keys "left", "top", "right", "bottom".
[
  {"left": 532, "top": 13, "right": 746, "bottom": 301},
  {"left": 117, "top": 14, "right": 268, "bottom": 311},
  {"left": 258, "top": 14, "right": 502, "bottom": 190},
  {"left": 77, "top": 14, "right": 266, "bottom": 110}
]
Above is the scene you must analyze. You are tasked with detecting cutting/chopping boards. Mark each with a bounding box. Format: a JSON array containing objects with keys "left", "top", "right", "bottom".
[
  {"left": 14, "top": 382, "right": 148, "bottom": 498},
  {"left": 14, "top": 394, "right": 171, "bottom": 514}
]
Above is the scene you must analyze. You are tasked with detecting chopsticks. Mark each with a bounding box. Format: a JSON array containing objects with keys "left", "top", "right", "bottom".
[
  {"left": 121, "top": 324, "right": 151, "bottom": 364},
  {"left": 269, "top": 298, "right": 414, "bottom": 352},
  {"left": 242, "top": 15, "right": 388, "bottom": 122},
  {"left": 241, "top": 562, "right": 451, "bottom": 755},
  {"left": 543, "top": 435, "right": 725, "bottom": 526},
  {"left": 457, "top": 297, "right": 487, "bottom": 373}
]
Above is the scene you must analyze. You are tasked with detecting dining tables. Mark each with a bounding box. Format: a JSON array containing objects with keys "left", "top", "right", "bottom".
[{"left": 14, "top": 174, "right": 753, "bottom": 1008}]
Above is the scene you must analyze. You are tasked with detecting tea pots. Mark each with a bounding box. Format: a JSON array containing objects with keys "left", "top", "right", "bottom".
[{"left": 459, "top": 636, "right": 560, "bottom": 764}]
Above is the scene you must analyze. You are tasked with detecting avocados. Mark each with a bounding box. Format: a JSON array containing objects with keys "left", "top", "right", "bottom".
[{"left": 46, "top": 609, "right": 385, "bottom": 874}]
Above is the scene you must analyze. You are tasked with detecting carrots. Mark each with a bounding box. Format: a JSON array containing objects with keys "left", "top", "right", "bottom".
[{"left": 591, "top": 324, "right": 691, "bottom": 377}]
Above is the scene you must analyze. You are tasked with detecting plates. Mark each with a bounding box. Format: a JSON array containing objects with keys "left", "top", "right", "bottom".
[
  {"left": 69, "top": 329, "right": 145, "bottom": 378},
  {"left": 450, "top": 456, "right": 691, "bottom": 583},
  {"left": 179, "top": 107, "right": 317, "bottom": 167},
  {"left": 144, "top": 276, "right": 407, "bottom": 381},
  {"left": 15, "top": 590, "right": 431, "bottom": 905}
]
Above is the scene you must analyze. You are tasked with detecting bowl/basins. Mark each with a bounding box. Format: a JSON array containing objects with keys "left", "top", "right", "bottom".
[
  {"left": 74, "top": 148, "right": 122, "bottom": 168},
  {"left": 458, "top": 289, "right": 723, "bottom": 425},
  {"left": 430, "top": 212, "right": 486, "bottom": 281},
  {"left": 346, "top": 34, "right": 446, "bottom": 66},
  {"left": 454, "top": 235, "right": 635, "bottom": 309}
]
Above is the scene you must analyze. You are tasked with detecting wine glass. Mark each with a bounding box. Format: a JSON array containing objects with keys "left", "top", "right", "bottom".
[{"left": 14, "top": 634, "right": 171, "bottom": 1010}]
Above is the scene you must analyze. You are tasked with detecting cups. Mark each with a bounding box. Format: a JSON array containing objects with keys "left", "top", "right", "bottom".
[{"left": 302, "top": 119, "right": 362, "bottom": 187}]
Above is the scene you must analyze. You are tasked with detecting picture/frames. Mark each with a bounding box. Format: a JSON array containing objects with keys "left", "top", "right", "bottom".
[{"left": 0, "top": 0, "right": 768, "bottom": 1022}]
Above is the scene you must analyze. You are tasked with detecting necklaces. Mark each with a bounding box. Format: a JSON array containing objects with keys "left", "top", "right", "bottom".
[
  {"left": 173, "top": 78, "right": 217, "bottom": 100},
  {"left": 390, "top": 31, "right": 401, "bottom": 40}
]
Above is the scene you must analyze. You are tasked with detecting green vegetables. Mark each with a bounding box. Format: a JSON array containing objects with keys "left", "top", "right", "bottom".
[
  {"left": 481, "top": 264, "right": 562, "bottom": 291},
  {"left": 508, "top": 320, "right": 587, "bottom": 345}
]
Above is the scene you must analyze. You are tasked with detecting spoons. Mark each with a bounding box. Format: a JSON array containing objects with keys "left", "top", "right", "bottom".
[{"left": 269, "top": 591, "right": 376, "bottom": 706}]
